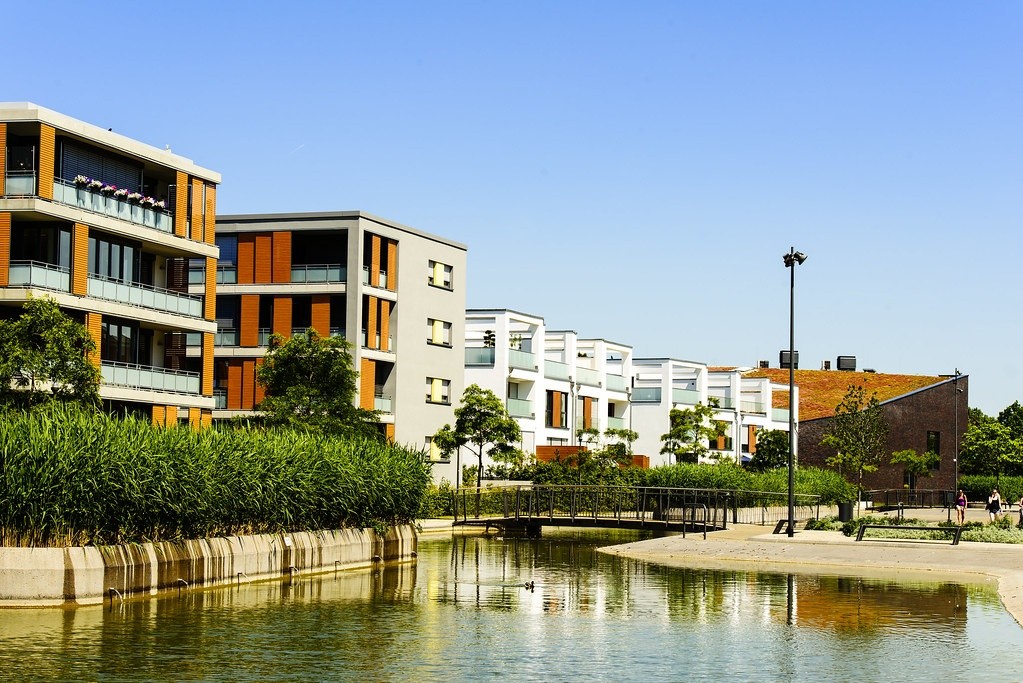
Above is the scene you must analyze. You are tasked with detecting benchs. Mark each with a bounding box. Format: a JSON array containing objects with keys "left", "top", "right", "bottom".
[
  {"left": 856, "top": 523, "right": 971, "bottom": 545},
  {"left": 772, "top": 519, "right": 798, "bottom": 533}
]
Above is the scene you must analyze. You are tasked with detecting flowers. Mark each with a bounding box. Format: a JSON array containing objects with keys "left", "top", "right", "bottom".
[{"left": 73, "top": 175, "right": 164, "bottom": 213}]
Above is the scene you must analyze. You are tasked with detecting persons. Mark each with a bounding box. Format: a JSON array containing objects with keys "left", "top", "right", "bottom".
[
  {"left": 955, "top": 489, "right": 967, "bottom": 526},
  {"left": 985, "top": 489, "right": 1003, "bottom": 524},
  {"left": 1019, "top": 498, "right": 1023, "bottom": 529}
]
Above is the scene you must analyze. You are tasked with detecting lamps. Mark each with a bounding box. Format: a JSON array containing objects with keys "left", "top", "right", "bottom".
[
  {"left": 160, "top": 263, "right": 166, "bottom": 270},
  {"left": 158, "top": 340, "right": 163, "bottom": 346}
]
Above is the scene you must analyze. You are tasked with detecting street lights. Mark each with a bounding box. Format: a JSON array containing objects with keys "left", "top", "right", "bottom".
[{"left": 782, "top": 247, "right": 809, "bottom": 538}]
